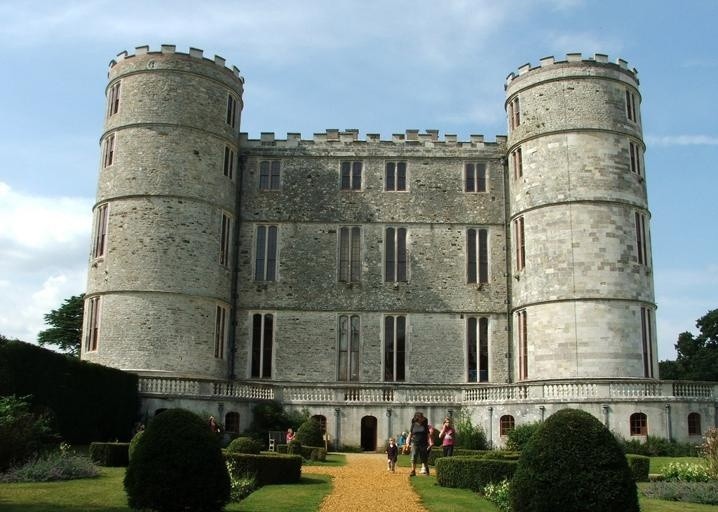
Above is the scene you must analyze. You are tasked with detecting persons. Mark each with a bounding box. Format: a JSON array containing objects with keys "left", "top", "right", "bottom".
[
  {"left": 208, "top": 416, "right": 220, "bottom": 433},
  {"left": 439, "top": 417, "right": 455, "bottom": 457},
  {"left": 384, "top": 413, "right": 435, "bottom": 476},
  {"left": 286, "top": 428, "right": 296, "bottom": 444}
]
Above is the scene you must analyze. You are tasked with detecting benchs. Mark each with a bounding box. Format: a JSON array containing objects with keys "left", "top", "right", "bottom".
[{"left": 269, "top": 431, "right": 288, "bottom": 451}]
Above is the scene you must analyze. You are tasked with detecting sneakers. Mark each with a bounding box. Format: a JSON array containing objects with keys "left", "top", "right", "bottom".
[
  {"left": 410, "top": 471, "right": 415, "bottom": 476},
  {"left": 419, "top": 467, "right": 430, "bottom": 475}
]
